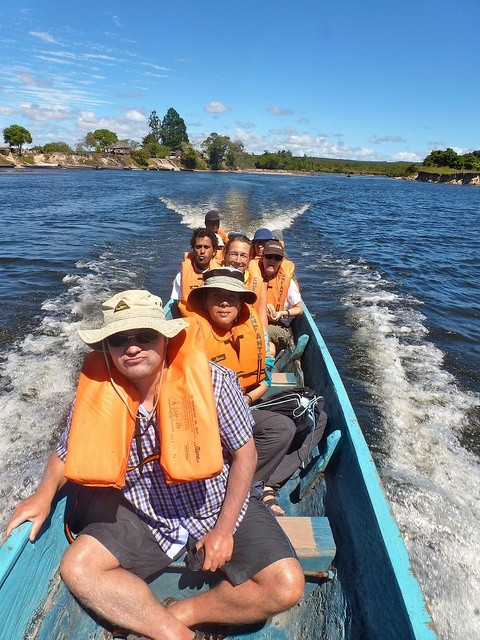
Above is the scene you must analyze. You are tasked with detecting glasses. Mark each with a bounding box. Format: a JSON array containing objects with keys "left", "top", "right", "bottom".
[
  {"left": 265, "top": 254, "right": 282, "bottom": 261},
  {"left": 108, "top": 331, "right": 158, "bottom": 347}
]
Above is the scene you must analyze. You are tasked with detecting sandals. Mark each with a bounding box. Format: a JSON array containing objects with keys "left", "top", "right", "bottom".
[{"left": 260, "top": 489, "right": 285, "bottom": 516}]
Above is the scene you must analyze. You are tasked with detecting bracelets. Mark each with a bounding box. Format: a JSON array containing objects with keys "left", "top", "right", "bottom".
[
  {"left": 283, "top": 309, "right": 290, "bottom": 320},
  {"left": 242, "top": 394, "right": 253, "bottom": 406}
]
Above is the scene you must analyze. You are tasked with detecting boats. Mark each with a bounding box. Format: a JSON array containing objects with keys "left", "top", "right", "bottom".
[{"left": 1, "top": 226, "right": 438, "bottom": 639}]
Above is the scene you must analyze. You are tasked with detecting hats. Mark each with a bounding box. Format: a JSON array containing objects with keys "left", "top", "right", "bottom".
[
  {"left": 77, "top": 290, "right": 188, "bottom": 352},
  {"left": 263, "top": 241, "right": 284, "bottom": 256},
  {"left": 205, "top": 210, "right": 221, "bottom": 221},
  {"left": 186, "top": 267, "right": 257, "bottom": 311},
  {"left": 252, "top": 229, "right": 274, "bottom": 240},
  {"left": 271, "top": 229, "right": 283, "bottom": 241}
]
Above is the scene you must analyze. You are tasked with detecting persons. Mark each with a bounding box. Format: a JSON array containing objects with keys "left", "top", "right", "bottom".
[
  {"left": 204, "top": 210, "right": 229, "bottom": 244},
  {"left": 222, "top": 234, "right": 268, "bottom": 336},
  {"left": 185, "top": 266, "right": 328, "bottom": 517},
  {"left": 1, "top": 288, "right": 306, "bottom": 640},
  {"left": 170, "top": 228, "right": 221, "bottom": 316},
  {"left": 253, "top": 228, "right": 300, "bottom": 292},
  {"left": 248, "top": 240, "right": 304, "bottom": 358}
]
